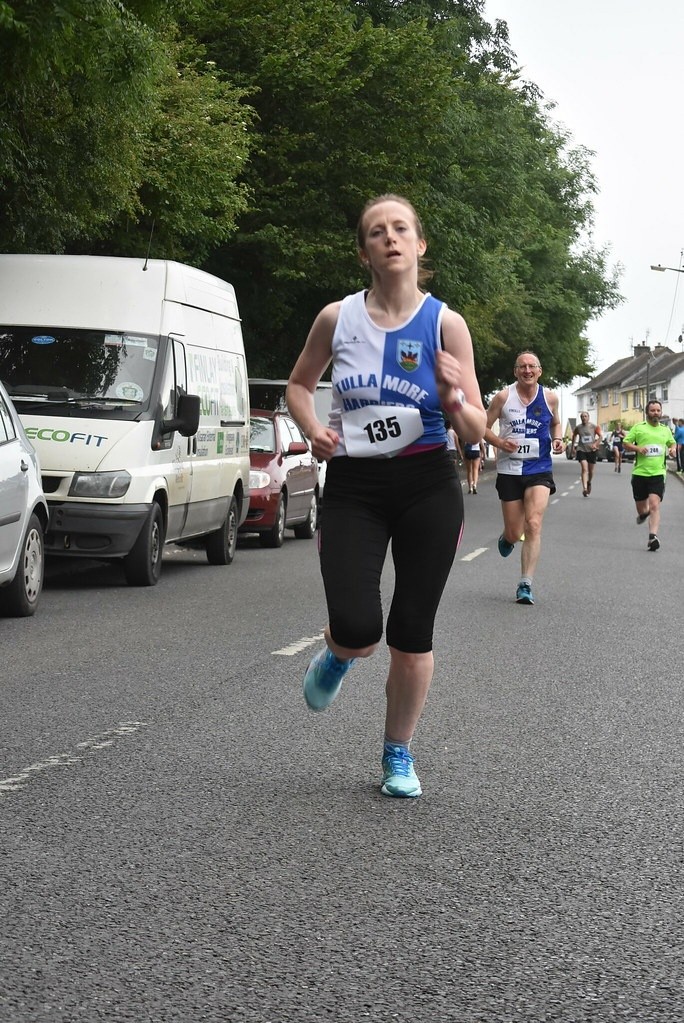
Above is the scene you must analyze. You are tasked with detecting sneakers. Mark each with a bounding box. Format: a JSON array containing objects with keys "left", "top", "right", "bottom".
[
  {"left": 636, "top": 513, "right": 650, "bottom": 525},
  {"left": 380, "top": 751, "right": 424, "bottom": 798},
  {"left": 499, "top": 533, "right": 516, "bottom": 558},
  {"left": 648, "top": 539, "right": 660, "bottom": 552},
  {"left": 304, "top": 647, "right": 358, "bottom": 712},
  {"left": 515, "top": 585, "right": 535, "bottom": 604}
]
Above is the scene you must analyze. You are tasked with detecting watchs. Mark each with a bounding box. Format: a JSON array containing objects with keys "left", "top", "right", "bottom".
[{"left": 443, "top": 388, "right": 465, "bottom": 414}]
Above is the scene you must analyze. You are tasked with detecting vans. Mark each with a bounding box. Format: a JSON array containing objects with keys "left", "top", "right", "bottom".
[{"left": 0, "top": 254, "right": 252, "bottom": 586}]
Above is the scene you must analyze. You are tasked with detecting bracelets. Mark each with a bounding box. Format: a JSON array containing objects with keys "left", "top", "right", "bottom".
[{"left": 552, "top": 438, "right": 563, "bottom": 442}]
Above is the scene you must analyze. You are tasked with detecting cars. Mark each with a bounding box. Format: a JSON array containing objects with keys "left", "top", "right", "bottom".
[
  {"left": 0, "top": 379, "right": 48, "bottom": 617},
  {"left": 599, "top": 431, "right": 636, "bottom": 463},
  {"left": 566, "top": 433, "right": 598, "bottom": 460},
  {"left": 176, "top": 407, "right": 321, "bottom": 548}
]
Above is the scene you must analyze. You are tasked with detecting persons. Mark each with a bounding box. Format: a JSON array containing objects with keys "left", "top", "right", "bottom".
[
  {"left": 286, "top": 195, "right": 487, "bottom": 799},
  {"left": 672, "top": 417, "right": 678, "bottom": 433},
  {"left": 609, "top": 422, "right": 626, "bottom": 473},
  {"left": 572, "top": 412, "right": 603, "bottom": 497},
  {"left": 622, "top": 401, "right": 677, "bottom": 551},
  {"left": 463, "top": 440, "right": 487, "bottom": 494},
  {"left": 674, "top": 418, "right": 684, "bottom": 475},
  {"left": 484, "top": 350, "right": 566, "bottom": 604},
  {"left": 444, "top": 420, "right": 464, "bottom": 470}
]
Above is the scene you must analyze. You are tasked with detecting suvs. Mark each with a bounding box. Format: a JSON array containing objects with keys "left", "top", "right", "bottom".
[{"left": 248, "top": 378, "right": 334, "bottom": 524}]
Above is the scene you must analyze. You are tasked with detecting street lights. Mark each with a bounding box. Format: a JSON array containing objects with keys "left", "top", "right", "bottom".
[{"left": 646, "top": 342, "right": 656, "bottom": 406}]
